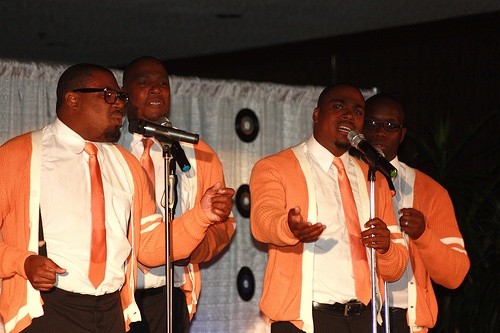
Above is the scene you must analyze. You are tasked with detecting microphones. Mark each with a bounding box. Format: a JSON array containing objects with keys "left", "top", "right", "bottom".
[
  {"left": 347, "top": 130, "right": 397, "bottom": 178},
  {"left": 375, "top": 148, "right": 396, "bottom": 197},
  {"left": 128, "top": 118, "right": 199, "bottom": 144},
  {"left": 157, "top": 116, "right": 191, "bottom": 173}
]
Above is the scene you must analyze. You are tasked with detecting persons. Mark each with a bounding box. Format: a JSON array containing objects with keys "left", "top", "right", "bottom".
[
  {"left": 364, "top": 93, "right": 471, "bottom": 333},
  {"left": 116, "top": 55, "right": 238, "bottom": 333},
  {"left": 250, "top": 83, "right": 410, "bottom": 333},
  {"left": 0, "top": 63, "right": 236, "bottom": 333}
]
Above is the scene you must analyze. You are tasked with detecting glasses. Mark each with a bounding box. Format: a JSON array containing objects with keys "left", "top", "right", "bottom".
[
  {"left": 70, "top": 88, "right": 130, "bottom": 105},
  {"left": 368, "top": 120, "right": 404, "bottom": 134}
]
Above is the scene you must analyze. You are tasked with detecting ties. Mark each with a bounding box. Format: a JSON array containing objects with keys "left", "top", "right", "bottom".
[
  {"left": 85, "top": 142, "right": 106, "bottom": 289},
  {"left": 139, "top": 139, "right": 156, "bottom": 190},
  {"left": 333, "top": 158, "right": 375, "bottom": 307}
]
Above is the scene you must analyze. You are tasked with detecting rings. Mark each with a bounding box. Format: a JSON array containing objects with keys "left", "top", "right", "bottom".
[{"left": 405, "top": 221, "right": 408, "bottom": 226}]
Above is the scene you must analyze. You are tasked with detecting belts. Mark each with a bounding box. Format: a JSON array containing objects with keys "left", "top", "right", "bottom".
[{"left": 313, "top": 300, "right": 370, "bottom": 316}]
